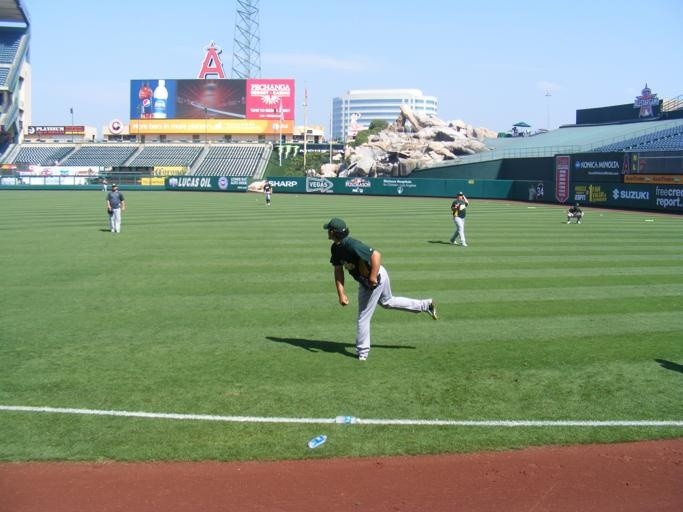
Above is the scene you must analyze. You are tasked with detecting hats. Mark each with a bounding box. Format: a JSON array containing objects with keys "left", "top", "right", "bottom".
[
  {"left": 324, "top": 218, "right": 346, "bottom": 232},
  {"left": 457, "top": 192, "right": 463, "bottom": 195},
  {"left": 112, "top": 183, "right": 116, "bottom": 187}
]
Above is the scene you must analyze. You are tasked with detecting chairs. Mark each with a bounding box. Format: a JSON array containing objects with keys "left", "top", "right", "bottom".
[
  {"left": 0, "top": 27, "right": 265, "bottom": 179},
  {"left": 434, "top": 117, "right": 681, "bottom": 168}
]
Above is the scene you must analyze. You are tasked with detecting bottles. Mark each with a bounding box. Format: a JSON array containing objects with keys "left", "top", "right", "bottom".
[
  {"left": 307, "top": 435, "right": 327, "bottom": 448},
  {"left": 336, "top": 415, "right": 360, "bottom": 424}
]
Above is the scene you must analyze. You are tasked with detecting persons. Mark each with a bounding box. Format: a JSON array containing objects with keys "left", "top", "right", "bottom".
[
  {"left": 321, "top": 218, "right": 439, "bottom": 361},
  {"left": 104, "top": 183, "right": 125, "bottom": 234},
  {"left": 102, "top": 178, "right": 108, "bottom": 192},
  {"left": 564, "top": 203, "right": 584, "bottom": 226},
  {"left": 447, "top": 191, "right": 469, "bottom": 248},
  {"left": 261, "top": 179, "right": 273, "bottom": 209}
]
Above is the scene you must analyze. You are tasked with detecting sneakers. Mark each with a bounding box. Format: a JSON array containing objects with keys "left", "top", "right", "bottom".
[
  {"left": 453, "top": 240, "right": 468, "bottom": 246},
  {"left": 111, "top": 229, "right": 120, "bottom": 232},
  {"left": 359, "top": 354, "right": 366, "bottom": 360},
  {"left": 426, "top": 303, "right": 438, "bottom": 320},
  {"left": 567, "top": 221, "right": 581, "bottom": 224}
]
